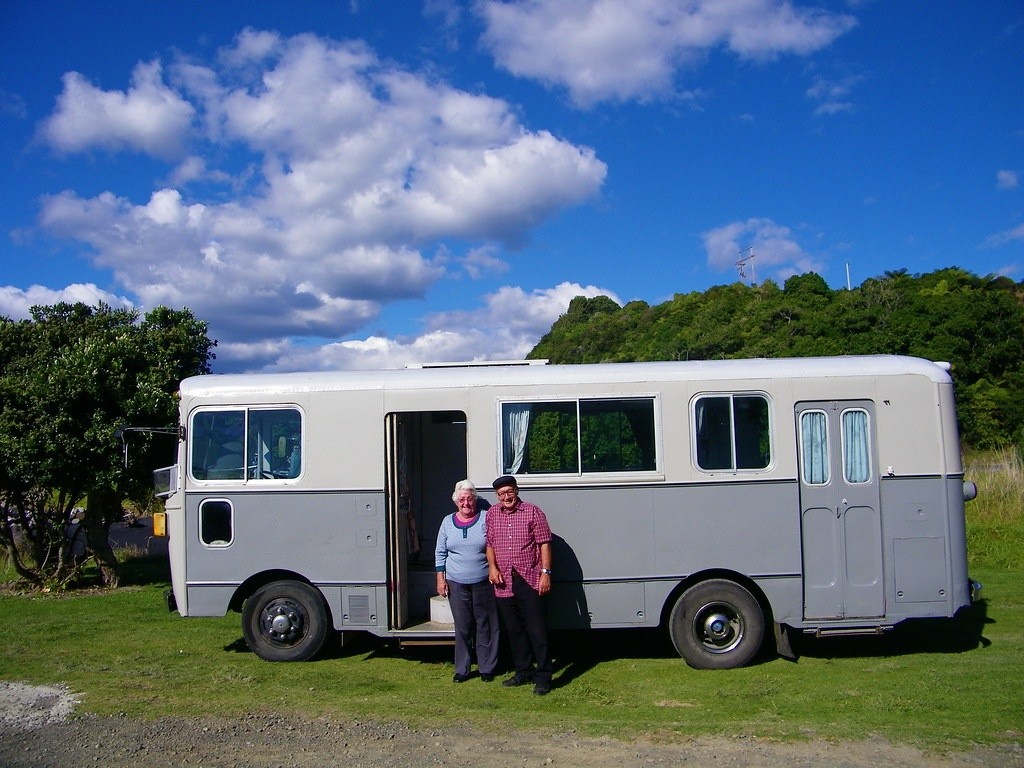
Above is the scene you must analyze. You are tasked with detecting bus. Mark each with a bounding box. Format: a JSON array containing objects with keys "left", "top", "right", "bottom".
[{"left": 120, "top": 353, "right": 982, "bottom": 671}]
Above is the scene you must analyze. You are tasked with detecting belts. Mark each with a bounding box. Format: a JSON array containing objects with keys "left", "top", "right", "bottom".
[{"left": 512, "top": 572, "right": 521, "bottom": 577}]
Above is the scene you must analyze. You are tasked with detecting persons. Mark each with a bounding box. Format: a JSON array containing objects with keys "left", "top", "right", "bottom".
[
  {"left": 487, "top": 476, "right": 554, "bottom": 695},
  {"left": 435, "top": 480, "right": 499, "bottom": 683}
]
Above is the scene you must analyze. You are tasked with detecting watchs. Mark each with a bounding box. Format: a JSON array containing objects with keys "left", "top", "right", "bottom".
[{"left": 542, "top": 568, "right": 551, "bottom": 575}]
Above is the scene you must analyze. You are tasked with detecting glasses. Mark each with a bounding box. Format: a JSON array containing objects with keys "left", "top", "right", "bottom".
[{"left": 496, "top": 489, "right": 517, "bottom": 497}]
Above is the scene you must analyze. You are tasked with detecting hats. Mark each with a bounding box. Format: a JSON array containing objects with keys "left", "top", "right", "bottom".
[{"left": 492, "top": 475, "right": 517, "bottom": 489}]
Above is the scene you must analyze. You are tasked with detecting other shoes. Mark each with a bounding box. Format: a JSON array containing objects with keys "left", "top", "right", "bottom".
[
  {"left": 453, "top": 672, "right": 466, "bottom": 683},
  {"left": 502, "top": 675, "right": 526, "bottom": 687},
  {"left": 480, "top": 673, "right": 494, "bottom": 682},
  {"left": 533, "top": 682, "right": 551, "bottom": 695}
]
